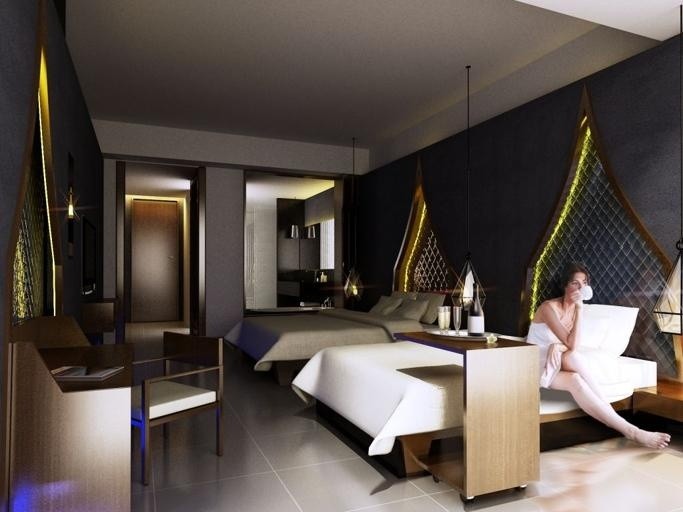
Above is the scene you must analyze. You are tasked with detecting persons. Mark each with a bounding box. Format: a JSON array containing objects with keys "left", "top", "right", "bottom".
[{"left": 527, "top": 263, "right": 671, "bottom": 450}]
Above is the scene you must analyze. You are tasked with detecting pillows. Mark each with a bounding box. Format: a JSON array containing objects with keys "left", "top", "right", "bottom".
[
  {"left": 585, "top": 303, "right": 639, "bottom": 355},
  {"left": 369, "top": 290, "right": 447, "bottom": 324}
]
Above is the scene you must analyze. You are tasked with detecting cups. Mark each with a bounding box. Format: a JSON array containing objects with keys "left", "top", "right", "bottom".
[{"left": 579, "top": 286, "right": 594, "bottom": 301}]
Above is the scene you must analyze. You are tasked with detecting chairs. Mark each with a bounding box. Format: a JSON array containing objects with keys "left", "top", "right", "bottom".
[
  {"left": 132, "top": 330, "right": 225, "bottom": 486},
  {"left": 79, "top": 298, "right": 124, "bottom": 345}
]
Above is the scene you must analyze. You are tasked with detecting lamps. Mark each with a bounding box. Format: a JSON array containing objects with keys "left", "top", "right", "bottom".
[
  {"left": 451, "top": 66, "right": 486, "bottom": 310},
  {"left": 65, "top": 185, "right": 76, "bottom": 220},
  {"left": 651, "top": 4, "right": 683, "bottom": 336},
  {"left": 343, "top": 137, "right": 364, "bottom": 301}
]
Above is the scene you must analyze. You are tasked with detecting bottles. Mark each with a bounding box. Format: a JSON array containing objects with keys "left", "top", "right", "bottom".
[
  {"left": 308, "top": 226, "right": 315, "bottom": 238},
  {"left": 468, "top": 283, "right": 485, "bottom": 335},
  {"left": 291, "top": 225, "right": 299, "bottom": 239}
]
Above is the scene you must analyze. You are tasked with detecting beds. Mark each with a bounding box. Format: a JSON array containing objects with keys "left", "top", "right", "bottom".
[
  {"left": 291, "top": 86, "right": 683, "bottom": 479},
  {"left": 223, "top": 150, "right": 475, "bottom": 387}
]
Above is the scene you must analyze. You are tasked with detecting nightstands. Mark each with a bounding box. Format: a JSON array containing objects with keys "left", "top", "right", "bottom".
[{"left": 632, "top": 382, "right": 683, "bottom": 431}]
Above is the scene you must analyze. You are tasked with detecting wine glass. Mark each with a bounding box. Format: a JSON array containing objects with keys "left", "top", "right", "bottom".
[{"left": 437, "top": 306, "right": 463, "bottom": 336}]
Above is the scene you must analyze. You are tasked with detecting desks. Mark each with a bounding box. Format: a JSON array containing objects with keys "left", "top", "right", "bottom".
[{"left": 8, "top": 314, "right": 135, "bottom": 512}]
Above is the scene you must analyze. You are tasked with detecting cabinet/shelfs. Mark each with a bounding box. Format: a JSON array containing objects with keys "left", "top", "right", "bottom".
[{"left": 394, "top": 329, "right": 539, "bottom": 504}]
